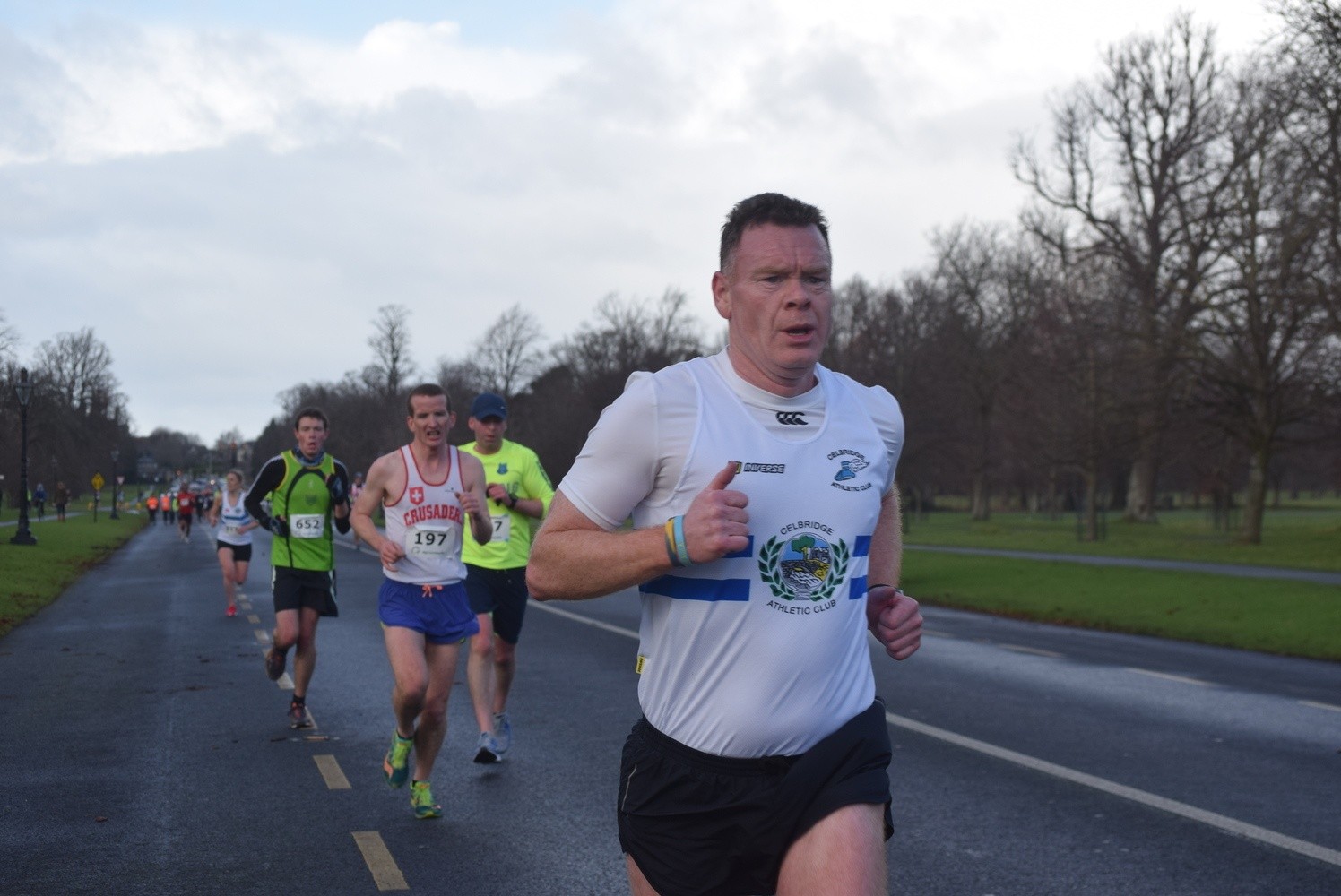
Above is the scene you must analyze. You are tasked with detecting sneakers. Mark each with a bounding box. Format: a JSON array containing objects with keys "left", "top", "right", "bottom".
[
  {"left": 288, "top": 702, "right": 313, "bottom": 729},
  {"left": 265, "top": 629, "right": 288, "bottom": 681},
  {"left": 473, "top": 731, "right": 501, "bottom": 763},
  {"left": 383, "top": 727, "right": 415, "bottom": 789},
  {"left": 489, "top": 708, "right": 511, "bottom": 753},
  {"left": 409, "top": 780, "right": 442, "bottom": 818}
]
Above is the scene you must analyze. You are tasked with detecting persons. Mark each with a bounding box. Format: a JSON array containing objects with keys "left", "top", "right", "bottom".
[
  {"left": 27, "top": 480, "right": 126, "bottom": 523},
  {"left": 244, "top": 408, "right": 352, "bottom": 733},
  {"left": 138, "top": 471, "right": 367, "bottom": 544},
  {"left": 209, "top": 469, "right": 260, "bottom": 617},
  {"left": 455, "top": 394, "right": 555, "bottom": 764},
  {"left": 525, "top": 192, "right": 923, "bottom": 896},
  {"left": 349, "top": 384, "right": 493, "bottom": 822}
]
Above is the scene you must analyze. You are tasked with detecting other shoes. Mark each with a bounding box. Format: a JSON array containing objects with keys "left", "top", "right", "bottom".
[{"left": 225, "top": 606, "right": 237, "bottom": 616}]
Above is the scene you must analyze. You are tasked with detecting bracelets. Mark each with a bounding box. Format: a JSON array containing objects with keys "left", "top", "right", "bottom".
[
  {"left": 665, "top": 515, "right": 696, "bottom": 569},
  {"left": 868, "top": 583, "right": 904, "bottom": 630}
]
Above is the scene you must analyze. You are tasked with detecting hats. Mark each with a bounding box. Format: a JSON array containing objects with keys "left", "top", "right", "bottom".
[{"left": 469, "top": 392, "right": 506, "bottom": 421}]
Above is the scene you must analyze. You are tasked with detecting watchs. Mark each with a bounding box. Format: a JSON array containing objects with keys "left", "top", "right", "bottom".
[{"left": 507, "top": 493, "right": 518, "bottom": 509}]
